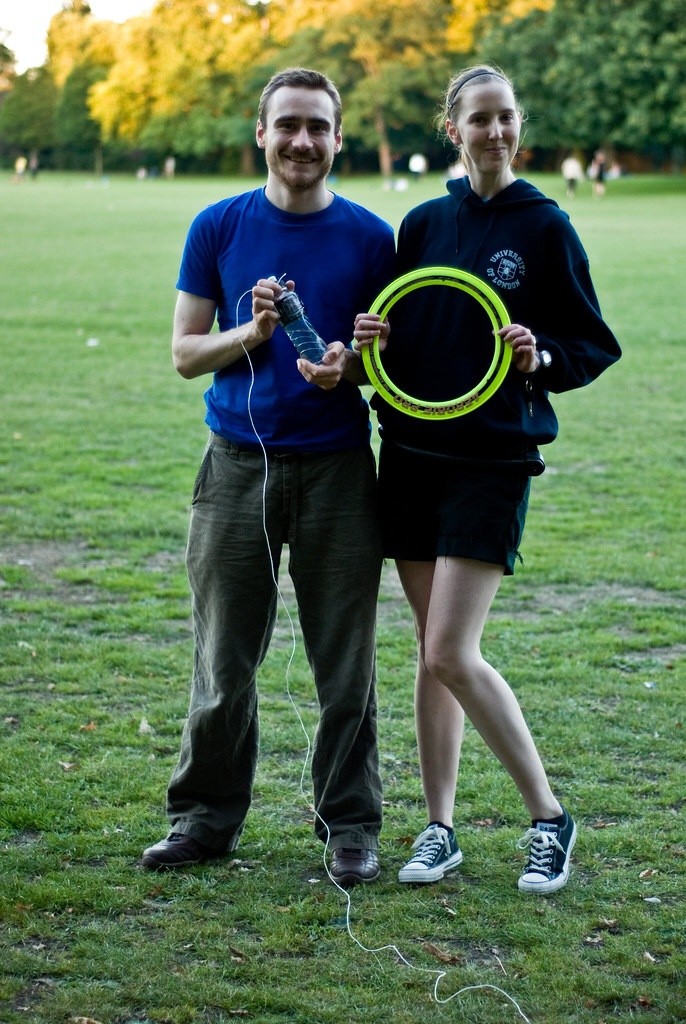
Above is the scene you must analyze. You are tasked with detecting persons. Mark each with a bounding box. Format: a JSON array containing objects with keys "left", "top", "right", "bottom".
[
  {"left": 349, "top": 65, "right": 623, "bottom": 895},
  {"left": 556, "top": 143, "right": 622, "bottom": 200},
  {"left": 140, "top": 65, "right": 398, "bottom": 884}
]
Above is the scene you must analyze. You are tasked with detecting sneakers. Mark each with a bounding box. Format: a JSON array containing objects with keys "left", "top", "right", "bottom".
[
  {"left": 398, "top": 823, "right": 462, "bottom": 883},
  {"left": 518, "top": 805, "right": 577, "bottom": 894}
]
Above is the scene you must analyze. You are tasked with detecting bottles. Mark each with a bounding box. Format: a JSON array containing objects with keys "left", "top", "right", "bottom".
[{"left": 267, "top": 276, "right": 331, "bottom": 367}]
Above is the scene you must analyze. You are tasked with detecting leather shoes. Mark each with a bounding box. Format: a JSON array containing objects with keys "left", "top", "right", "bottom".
[
  {"left": 330, "top": 849, "right": 381, "bottom": 882},
  {"left": 143, "top": 832, "right": 208, "bottom": 867}
]
